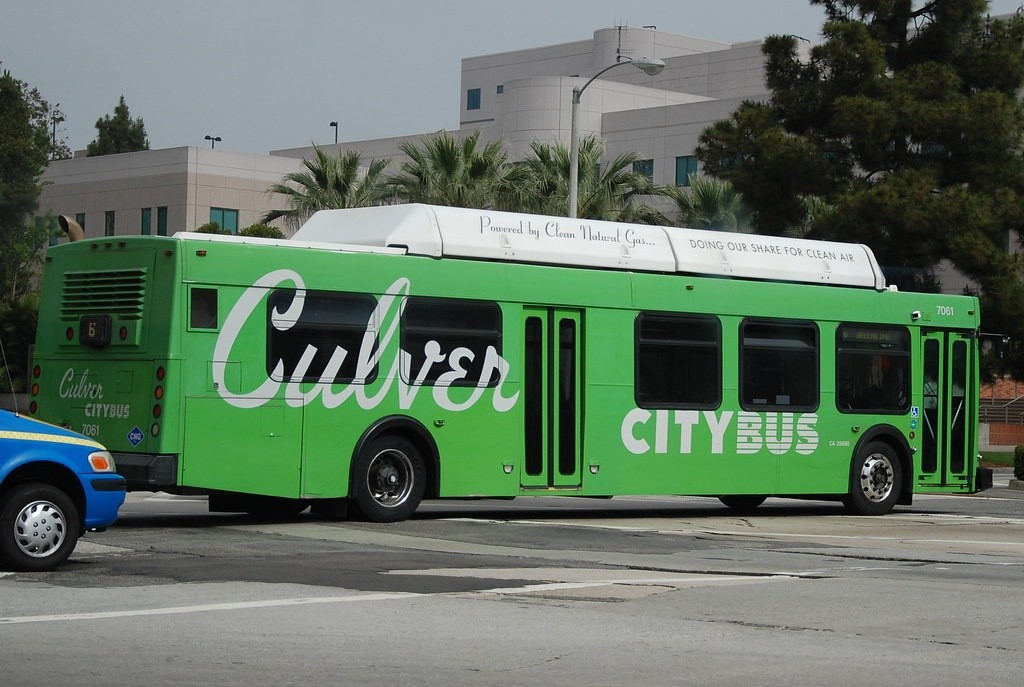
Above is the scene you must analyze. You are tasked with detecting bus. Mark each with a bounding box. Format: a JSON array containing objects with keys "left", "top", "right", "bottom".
[{"left": 25, "top": 203, "right": 993, "bottom": 521}]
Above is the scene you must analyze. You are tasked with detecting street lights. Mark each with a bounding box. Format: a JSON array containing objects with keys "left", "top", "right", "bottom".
[{"left": 563, "top": 50, "right": 671, "bottom": 219}]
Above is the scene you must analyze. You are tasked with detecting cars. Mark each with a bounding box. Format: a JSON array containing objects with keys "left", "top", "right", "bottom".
[{"left": 0, "top": 406, "right": 125, "bottom": 573}]
